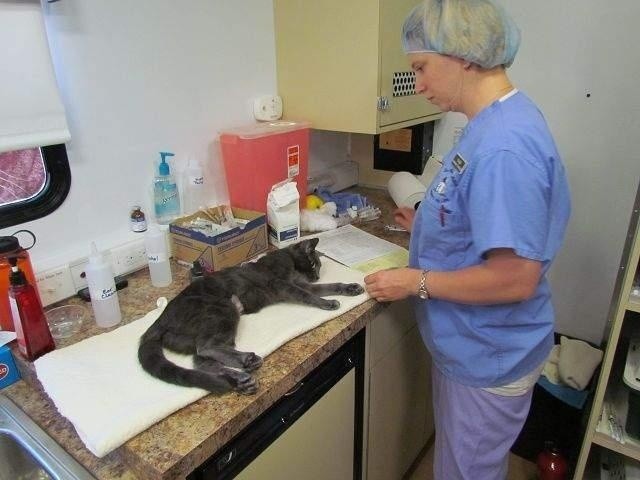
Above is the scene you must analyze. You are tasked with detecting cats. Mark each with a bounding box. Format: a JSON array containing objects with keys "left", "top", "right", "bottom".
[{"left": 137, "top": 237, "right": 365, "bottom": 397}]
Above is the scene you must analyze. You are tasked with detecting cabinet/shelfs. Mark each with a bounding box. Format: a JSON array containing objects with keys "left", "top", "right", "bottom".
[{"left": 572, "top": 215, "right": 639, "bottom": 479}]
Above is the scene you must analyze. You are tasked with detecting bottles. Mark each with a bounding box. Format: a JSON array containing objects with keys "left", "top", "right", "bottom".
[
  {"left": 145, "top": 221, "right": 173, "bottom": 289},
  {"left": 131, "top": 206, "right": 147, "bottom": 232},
  {"left": 84, "top": 240, "right": 123, "bottom": 328},
  {"left": 0, "top": 229, "right": 44, "bottom": 342},
  {"left": 182, "top": 157, "right": 208, "bottom": 216}
]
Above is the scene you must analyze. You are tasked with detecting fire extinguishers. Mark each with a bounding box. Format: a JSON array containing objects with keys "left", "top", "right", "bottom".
[{"left": 536, "top": 439, "right": 568, "bottom": 480}]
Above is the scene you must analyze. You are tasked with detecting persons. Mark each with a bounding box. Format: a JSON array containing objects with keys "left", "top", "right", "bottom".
[{"left": 362, "top": 1, "right": 573, "bottom": 479}]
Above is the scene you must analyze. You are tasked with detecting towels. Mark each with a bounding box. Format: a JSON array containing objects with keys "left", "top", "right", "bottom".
[
  {"left": 541, "top": 335, "right": 604, "bottom": 392},
  {"left": 32, "top": 254, "right": 375, "bottom": 460}
]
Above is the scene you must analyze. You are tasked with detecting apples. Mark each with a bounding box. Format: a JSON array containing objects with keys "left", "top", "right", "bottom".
[{"left": 305, "top": 194, "right": 324, "bottom": 211}]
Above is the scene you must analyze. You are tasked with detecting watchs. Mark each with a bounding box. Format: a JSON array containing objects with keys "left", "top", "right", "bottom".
[{"left": 418, "top": 269, "right": 430, "bottom": 301}]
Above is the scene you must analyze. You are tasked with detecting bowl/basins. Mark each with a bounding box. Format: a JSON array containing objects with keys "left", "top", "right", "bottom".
[{"left": 43, "top": 305, "right": 87, "bottom": 339}]
